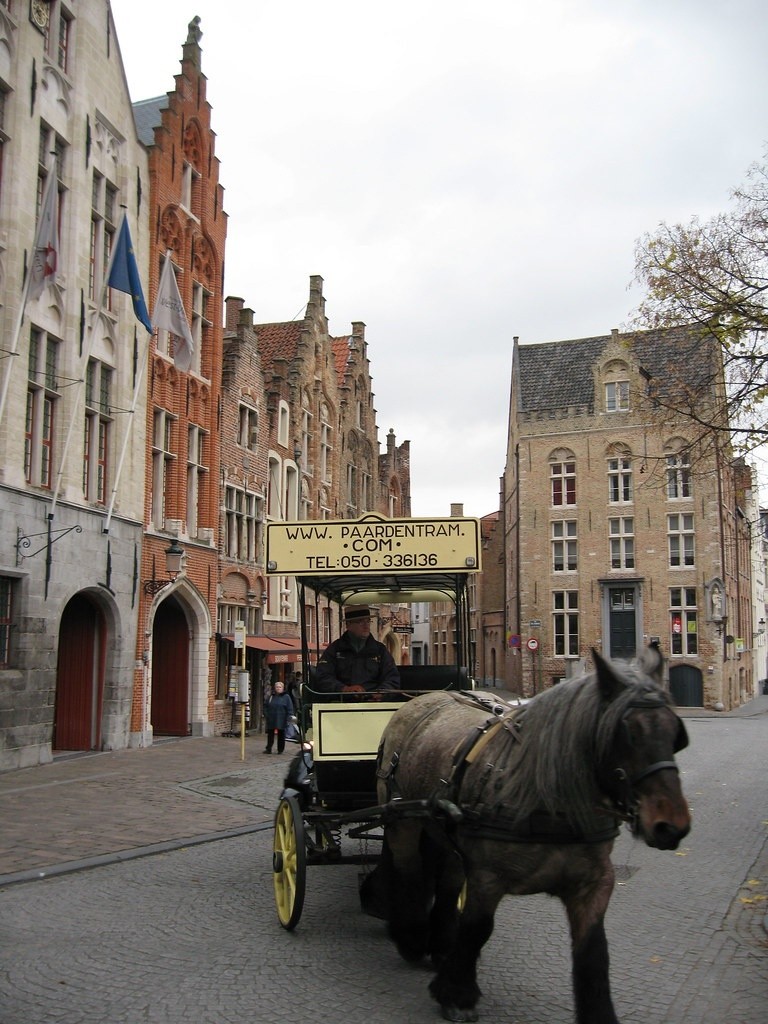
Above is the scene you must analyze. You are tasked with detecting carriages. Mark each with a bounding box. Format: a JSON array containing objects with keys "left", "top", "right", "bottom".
[{"left": 261, "top": 503, "right": 692, "bottom": 1024}]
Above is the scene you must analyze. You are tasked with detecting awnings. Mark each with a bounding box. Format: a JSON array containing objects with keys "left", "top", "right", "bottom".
[{"left": 222, "top": 635, "right": 328, "bottom": 665}]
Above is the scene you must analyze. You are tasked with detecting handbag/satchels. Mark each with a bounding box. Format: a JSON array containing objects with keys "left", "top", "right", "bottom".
[{"left": 285, "top": 721, "right": 300, "bottom": 741}]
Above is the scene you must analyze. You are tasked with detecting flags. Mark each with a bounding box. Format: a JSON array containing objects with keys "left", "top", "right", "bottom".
[
  {"left": 153, "top": 251, "right": 194, "bottom": 375},
  {"left": 105, "top": 211, "right": 156, "bottom": 336},
  {"left": 23, "top": 156, "right": 67, "bottom": 333}
]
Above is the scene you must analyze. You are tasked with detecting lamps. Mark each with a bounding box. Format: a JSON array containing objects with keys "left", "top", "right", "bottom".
[
  {"left": 258, "top": 590, "right": 267, "bottom": 605},
  {"left": 144, "top": 538, "right": 185, "bottom": 594},
  {"left": 650, "top": 636, "right": 660, "bottom": 643},
  {"left": 246, "top": 590, "right": 257, "bottom": 602}
]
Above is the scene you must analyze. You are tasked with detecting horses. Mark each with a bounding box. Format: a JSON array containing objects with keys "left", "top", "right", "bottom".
[{"left": 360, "top": 638, "right": 693, "bottom": 1024}]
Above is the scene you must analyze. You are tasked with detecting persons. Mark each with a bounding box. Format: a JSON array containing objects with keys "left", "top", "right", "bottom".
[
  {"left": 315, "top": 604, "right": 400, "bottom": 702},
  {"left": 263, "top": 682, "right": 294, "bottom": 755},
  {"left": 290, "top": 672, "right": 303, "bottom": 715}
]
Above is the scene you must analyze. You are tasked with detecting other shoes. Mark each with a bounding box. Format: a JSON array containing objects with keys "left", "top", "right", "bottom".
[
  {"left": 278, "top": 751, "right": 282, "bottom": 754},
  {"left": 263, "top": 750, "right": 272, "bottom": 754}
]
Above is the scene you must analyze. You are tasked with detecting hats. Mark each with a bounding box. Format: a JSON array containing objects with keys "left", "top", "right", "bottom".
[{"left": 338, "top": 604, "right": 379, "bottom": 622}]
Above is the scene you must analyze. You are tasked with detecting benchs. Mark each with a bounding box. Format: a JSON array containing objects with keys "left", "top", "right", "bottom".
[{"left": 313, "top": 664, "right": 467, "bottom": 762}]
